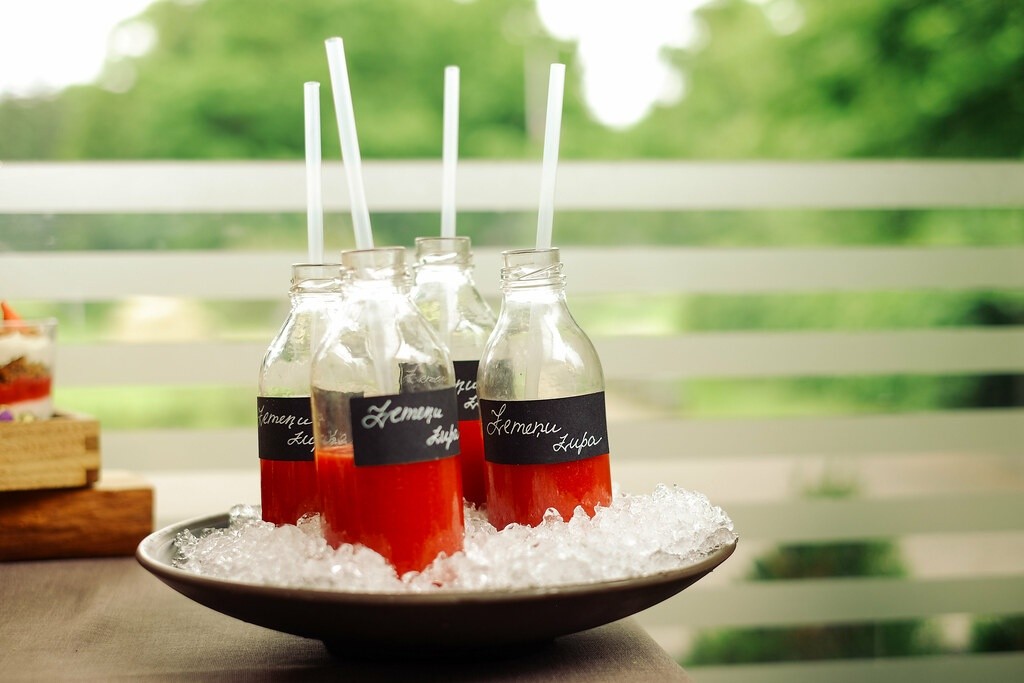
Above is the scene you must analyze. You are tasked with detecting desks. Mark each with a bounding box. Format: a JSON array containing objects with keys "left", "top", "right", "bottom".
[{"left": 0, "top": 546, "right": 692, "bottom": 683}]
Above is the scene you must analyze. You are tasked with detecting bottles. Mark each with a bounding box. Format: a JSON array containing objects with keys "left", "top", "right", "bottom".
[
  {"left": 256, "top": 254, "right": 346, "bottom": 530},
  {"left": 309, "top": 245, "right": 467, "bottom": 581},
  {"left": 408, "top": 232, "right": 498, "bottom": 510},
  {"left": 476, "top": 245, "right": 614, "bottom": 531}
]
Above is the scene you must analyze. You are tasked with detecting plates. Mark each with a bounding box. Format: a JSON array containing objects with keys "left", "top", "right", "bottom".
[{"left": 133, "top": 504, "right": 738, "bottom": 642}]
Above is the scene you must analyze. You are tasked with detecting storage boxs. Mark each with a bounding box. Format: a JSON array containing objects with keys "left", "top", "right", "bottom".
[{"left": 1, "top": 405, "right": 156, "bottom": 555}]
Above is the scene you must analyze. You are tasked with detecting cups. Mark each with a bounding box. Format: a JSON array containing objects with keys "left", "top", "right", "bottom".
[{"left": 1, "top": 318, "right": 56, "bottom": 422}]
